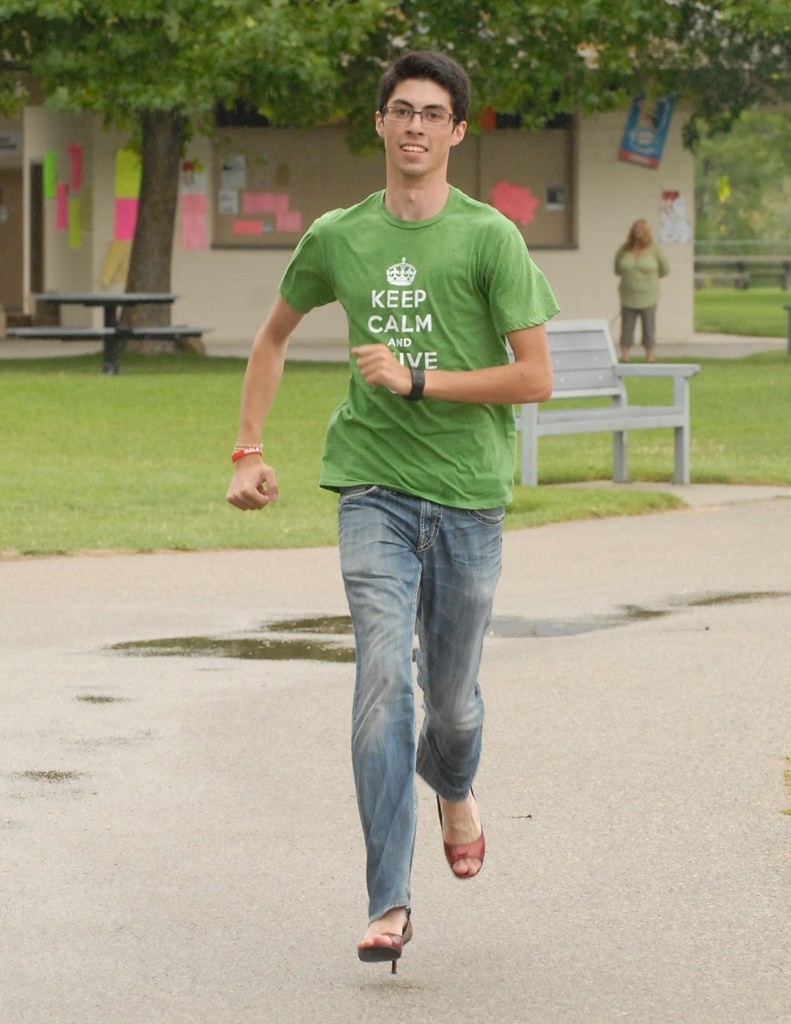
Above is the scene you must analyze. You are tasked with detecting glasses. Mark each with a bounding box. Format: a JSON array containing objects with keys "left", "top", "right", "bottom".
[{"left": 385, "top": 105, "right": 459, "bottom": 127}]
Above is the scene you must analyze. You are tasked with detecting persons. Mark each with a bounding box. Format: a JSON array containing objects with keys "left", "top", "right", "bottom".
[
  {"left": 224, "top": 48, "right": 561, "bottom": 978},
  {"left": 613, "top": 218, "right": 670, "bottom": 362}
]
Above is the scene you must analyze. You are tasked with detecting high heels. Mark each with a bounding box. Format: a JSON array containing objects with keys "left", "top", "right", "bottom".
[
  {"left": 359, "top": 908, "right": 412, "bottom": 974},
  {"left": 436, "top": 786, "right": 490, "bottom": 880}
]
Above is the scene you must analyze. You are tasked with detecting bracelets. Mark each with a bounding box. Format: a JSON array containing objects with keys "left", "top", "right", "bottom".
[
  {"left": 235, "top": 444, "right": 263, "bottom": 451},
  {"left": 402, "top": 366, "right": 426, "bottom": 402},
  {"left": 231, "top": 447, "right": 263, "bottom": 463}
]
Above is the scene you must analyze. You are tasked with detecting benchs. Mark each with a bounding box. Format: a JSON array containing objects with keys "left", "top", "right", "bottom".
[
  {"left": 505, "top": 318, "right": 701, "bottom": 488},
  {"left": 5, "top": 291, "right": 206, "bottom": 375}
]
